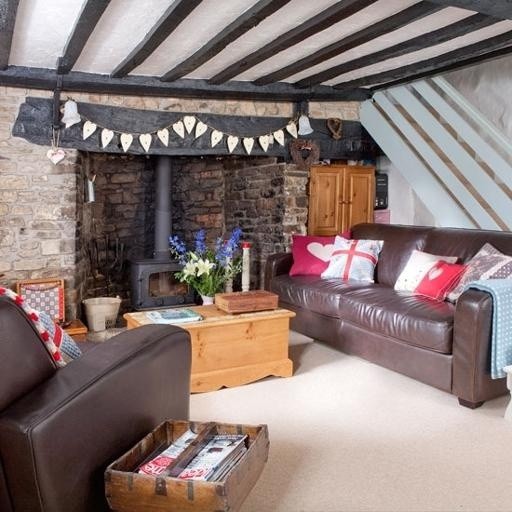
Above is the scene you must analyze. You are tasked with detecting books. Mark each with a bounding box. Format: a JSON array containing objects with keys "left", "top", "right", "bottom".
[
  {"left": 145, "top": 306, "right": 203, "bottom": 324},
  {"left": 139, "top": 428, "right": 248, "bottom": 482}
]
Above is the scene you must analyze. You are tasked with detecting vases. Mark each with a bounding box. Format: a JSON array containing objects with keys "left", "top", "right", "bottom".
[{"left": 198, "top": 292, "right": 215, "bottom": 307}]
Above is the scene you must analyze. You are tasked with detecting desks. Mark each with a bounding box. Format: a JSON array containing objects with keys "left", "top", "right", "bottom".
[
  {"left": 123, "top": 299, "right": 298, "bottom": 395},
  {"left": 51, "top": 317, "right": 87, "bottom": 342}
]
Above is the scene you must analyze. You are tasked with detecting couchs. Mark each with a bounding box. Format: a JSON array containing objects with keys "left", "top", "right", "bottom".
[
  {"left": 262, "top": 219, "right": 512, "bottom": 410},
  {"left": 0, "top": 293, "right": 192, "bottom": 510}
]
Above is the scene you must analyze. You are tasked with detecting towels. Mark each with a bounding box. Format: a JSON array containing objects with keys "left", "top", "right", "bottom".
[{"left": 461, "top": 274, "right": 511, "bottom": 379}]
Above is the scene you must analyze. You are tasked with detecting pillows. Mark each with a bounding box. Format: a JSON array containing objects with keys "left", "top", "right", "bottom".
[
  {"left": 322, "top": 232, "right": 384, "bottom": 285},
  {"left": 393, "top": 247, "right": 457, "bottom": 296},
  {"left": 288, "top": 230, "right": 352, "bottom": 278},
  {"left": 448, "top": 241, "right": 512, "bottom": 303},
  {"left": 418, "top": 259, "right": 471, "bottom": 302}
]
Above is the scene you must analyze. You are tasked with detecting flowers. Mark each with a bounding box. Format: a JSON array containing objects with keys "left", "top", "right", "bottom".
[{"left": 167, "top": 224, "right": 248, "bottom": 294}]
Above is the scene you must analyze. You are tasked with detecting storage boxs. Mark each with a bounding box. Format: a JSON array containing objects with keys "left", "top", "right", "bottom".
[
  {"left": 215, "top": 289, "right": 279, "bottom": 313},
  {"left": 102, "top": 414, "right": 269, "bottom": 512}
]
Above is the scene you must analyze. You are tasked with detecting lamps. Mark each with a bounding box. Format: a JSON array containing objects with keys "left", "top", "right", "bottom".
[
  {"left": 294, "top": 94, "right": 315, "bottom": 137},
  {"left": 49, "top": 88, "right": 81, "bottom": 131}
]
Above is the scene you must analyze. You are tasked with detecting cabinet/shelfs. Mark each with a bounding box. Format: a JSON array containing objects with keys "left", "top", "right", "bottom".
[{"left": 307, "top": 162, "right": 376, "bottom": 236}]
